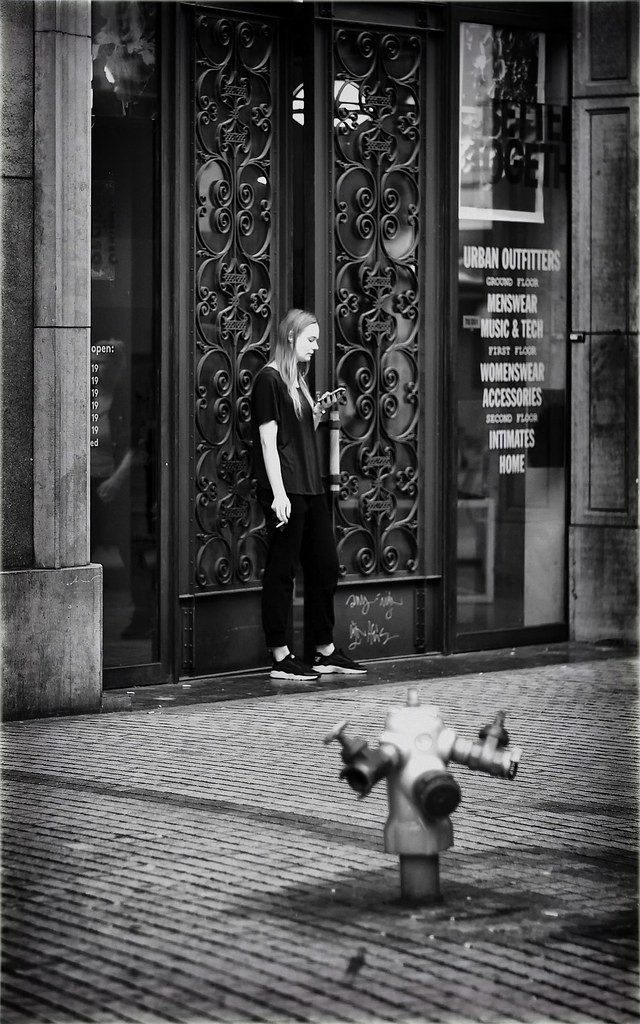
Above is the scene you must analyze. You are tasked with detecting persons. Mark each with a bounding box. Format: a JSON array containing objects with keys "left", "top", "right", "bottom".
[{"left": 249, "top": 309, "right": 368, "bottom": 681}]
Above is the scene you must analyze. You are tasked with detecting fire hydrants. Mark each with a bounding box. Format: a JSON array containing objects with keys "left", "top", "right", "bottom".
[{"left": 321, "top": 687, "right": 523, "bottom": 905}]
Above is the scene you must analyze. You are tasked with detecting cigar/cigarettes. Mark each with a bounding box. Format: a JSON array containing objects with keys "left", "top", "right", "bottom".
[{"left": 276, "top": 520, "right": 286, "bottom": 528}]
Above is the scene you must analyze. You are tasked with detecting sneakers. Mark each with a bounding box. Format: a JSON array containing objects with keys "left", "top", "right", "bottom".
[
  {"left": 269, "top": 653, "right": 322, "bottom": 680},
  {"left": 311, "top": 647, "right": 368, "bottom": 674}
]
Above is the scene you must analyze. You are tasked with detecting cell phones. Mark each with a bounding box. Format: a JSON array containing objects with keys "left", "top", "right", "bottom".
[{"left": 318, "top": 387, "right": 346, "bottom": 405}]
